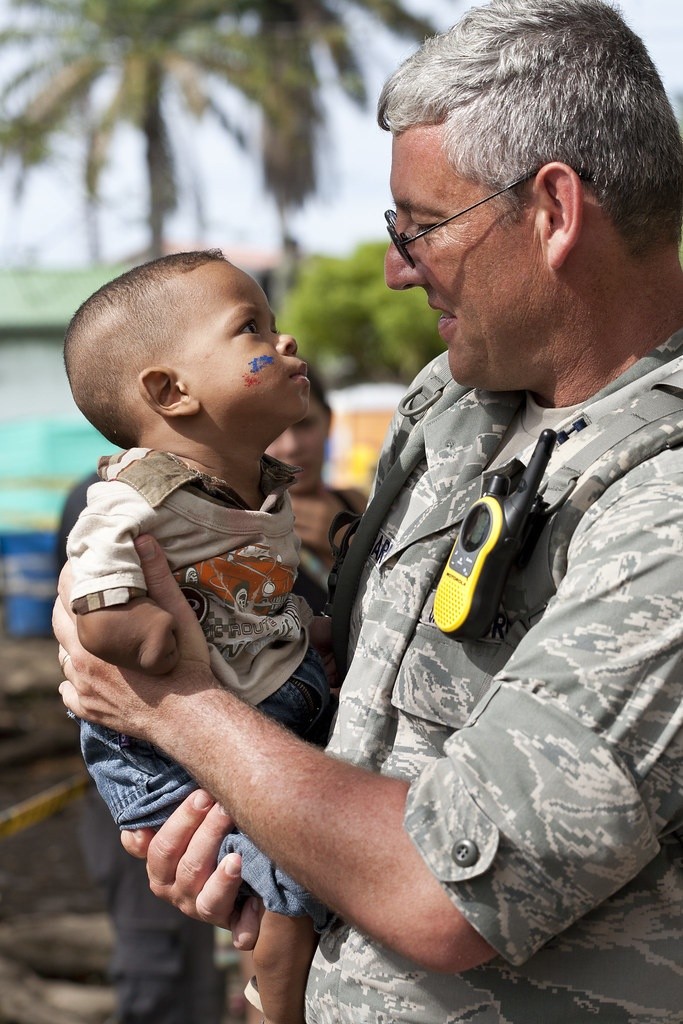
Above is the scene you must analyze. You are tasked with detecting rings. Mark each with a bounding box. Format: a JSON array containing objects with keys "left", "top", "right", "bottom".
[{"left": 61, "top": 653, "right": 70, "bottom": 678}]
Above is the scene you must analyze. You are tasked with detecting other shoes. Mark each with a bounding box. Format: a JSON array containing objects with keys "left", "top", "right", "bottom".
[{"left": 244, "top": 975, "right": 264, "bottom": 1013}]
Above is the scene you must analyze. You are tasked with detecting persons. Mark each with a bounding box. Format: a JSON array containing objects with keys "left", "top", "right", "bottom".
[
  {"left": 51, "top": 0, "right": 683, "bottom": 1024},
  {"left": 57, "top": 364, "right": 372, "bottom": 1024},
  {"left": 65, "top": 252, "right": 341, "bottom": 1024}
]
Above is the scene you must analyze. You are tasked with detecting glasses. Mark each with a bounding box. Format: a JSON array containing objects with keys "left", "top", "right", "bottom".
[{"left": 384, "top": 170, "right": 595, "bottom": 269}]
